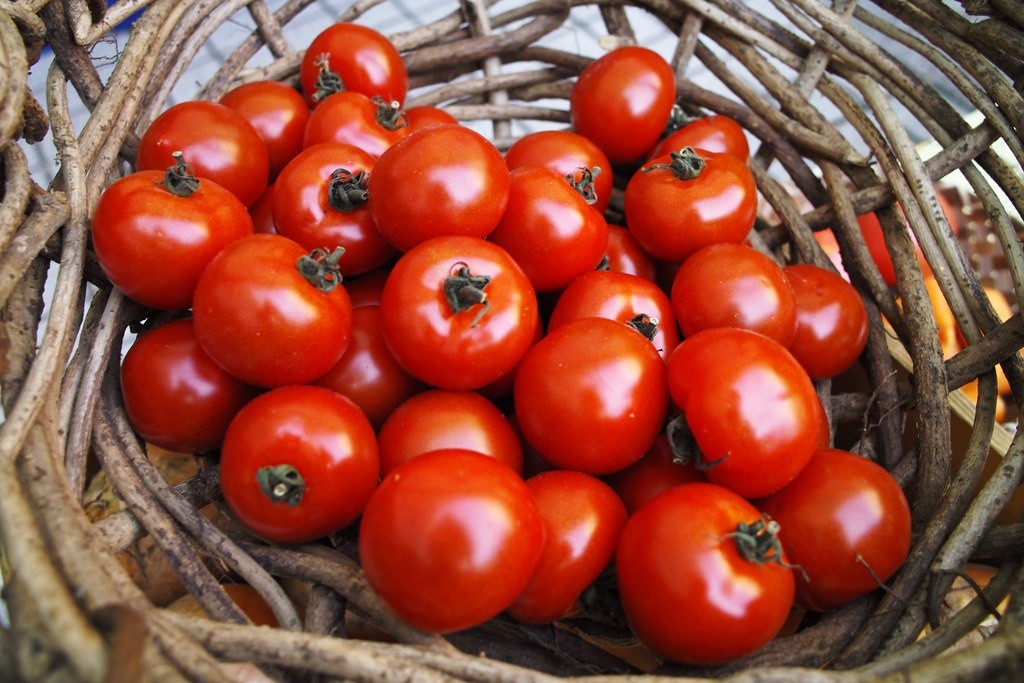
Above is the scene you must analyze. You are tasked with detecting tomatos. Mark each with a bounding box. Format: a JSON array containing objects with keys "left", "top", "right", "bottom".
[{"left": 92, "top": 24, "right": 912, "bottom": 664}]
